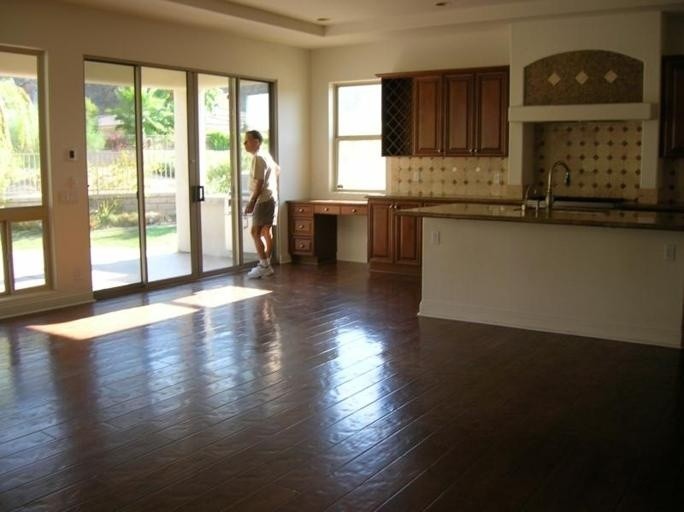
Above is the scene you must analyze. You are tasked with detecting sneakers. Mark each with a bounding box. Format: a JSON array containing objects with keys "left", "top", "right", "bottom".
[{"left": 247, "top": 262, "right": 274, "bottom": 278}]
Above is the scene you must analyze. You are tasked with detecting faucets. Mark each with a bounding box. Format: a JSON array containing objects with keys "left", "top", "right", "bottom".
[
  {"left": 544, "top": 160, "right": 572, "bottom": 207},
  {"left": 524, "top": 184, "right": 538, "bottom": 204}
]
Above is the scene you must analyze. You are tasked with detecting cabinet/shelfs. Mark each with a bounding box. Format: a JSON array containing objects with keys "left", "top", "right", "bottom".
[
  {"left": 411, "top": 73, "right": 445, "bottom": 157},
  {"left": 286, "top": 199, "right": 369, "bottom": 266},
  {"left": 373, "top": 70, "right": 412, "bottom": 157},
  {"left": 366, "top": 197, "right": 423, "bottom": 276},
  {"left": 445, "top": 69, "right": 510, "bottom": 158}
]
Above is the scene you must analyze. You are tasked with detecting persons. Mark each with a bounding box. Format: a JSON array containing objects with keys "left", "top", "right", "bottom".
[{"left": 243, "top": 130, "right": 281, "bottom": 278}]
